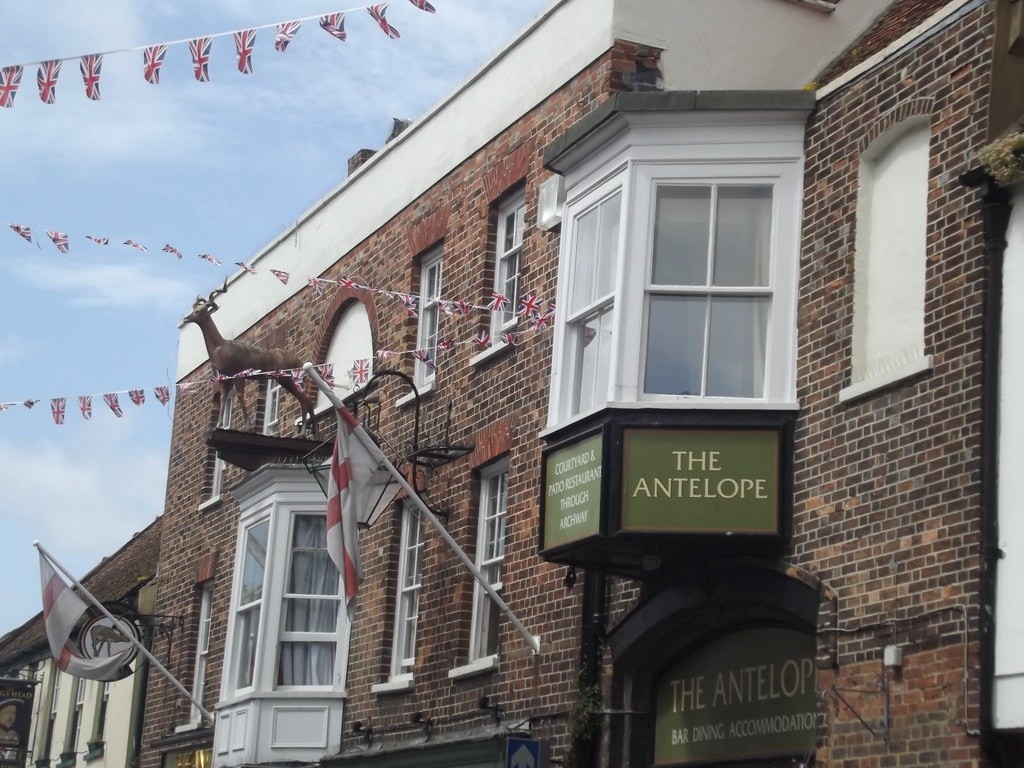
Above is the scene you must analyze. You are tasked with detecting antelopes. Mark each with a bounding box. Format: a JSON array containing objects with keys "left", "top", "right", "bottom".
[{"left": 175, "top": 273, "right": 328, "bottom": 445}]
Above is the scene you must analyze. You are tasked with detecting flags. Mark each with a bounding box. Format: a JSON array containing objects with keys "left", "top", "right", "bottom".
[
  {"left": 9, "top": 224, "right": 554, "bottom": 320},
  {"left": 0, "top": 0, "right": 436, "bottom": 109},
  {"left": 328, "top": 393, "right": 408, "bottom": 615},
  {"left": 36, "top": 552, "right": 139, "bottom": 682},
  {"left": 0, "top": 319, "right": 556, "bottom": 425}
]
